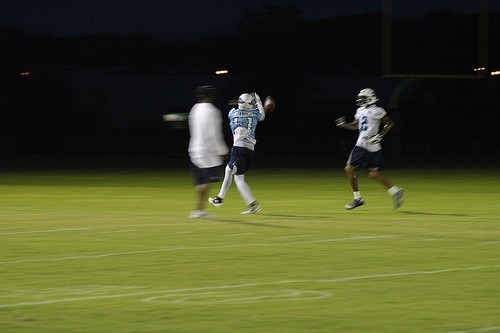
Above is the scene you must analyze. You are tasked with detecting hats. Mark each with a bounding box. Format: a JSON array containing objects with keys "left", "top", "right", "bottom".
[{"left": 195, "top": 85, "right": 218, "bottom": 98}]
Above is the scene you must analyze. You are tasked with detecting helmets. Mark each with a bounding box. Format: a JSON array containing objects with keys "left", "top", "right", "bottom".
[
  {"left": 237, "top": 93, "right": 258, "bottom": 111},
  {"left": 356, "top": 87, "right": 380, "bottom": 107}
]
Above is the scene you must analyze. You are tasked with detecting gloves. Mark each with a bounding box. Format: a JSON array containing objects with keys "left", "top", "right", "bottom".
[
  {"left": 254, "top": 92, "right": 261, "bottom": 104},
  {"left": 368, "top": 133, "right": 382, "bottom": 145},
  {"left": 335, "top": 116, "right": 347, "bottom": 129}
]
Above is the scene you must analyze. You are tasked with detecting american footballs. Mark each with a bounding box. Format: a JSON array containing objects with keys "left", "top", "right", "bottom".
[{"left": 264, "top": 96, "right": 275, "bottom": 114}]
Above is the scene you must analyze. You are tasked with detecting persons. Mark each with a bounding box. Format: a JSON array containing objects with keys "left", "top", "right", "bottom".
[
  {"left": 208, "top": 94, "right": 265, "bottom": 215},
  {"left": 189, "top": 87, "right": 229, "bottom": 217},
  {"left": 336, "top": 88, "right": 403, "bottom": 210}
]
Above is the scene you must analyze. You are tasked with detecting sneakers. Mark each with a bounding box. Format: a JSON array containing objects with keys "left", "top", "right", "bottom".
[
  {"left": 240, "top": 204, "right": 262, "bottom": 215},
  {"left": 344, "top": 196, "right": 365, "bottom": 210},
  {"left": 392, "top": 188, "right": 409, "bottom": 209},
  {"left": 188, "top": 209, "right": 216, "bottom": 219},
  {"left": 208, "top": 195, "right": 224, "bottom": 207}
]
